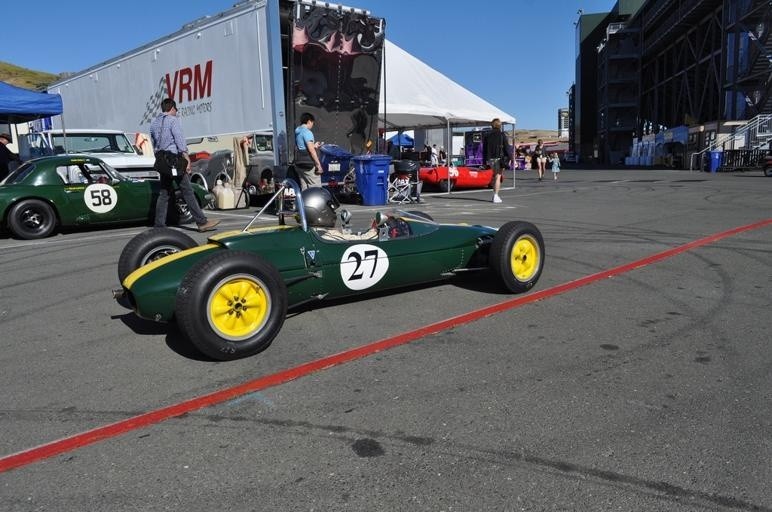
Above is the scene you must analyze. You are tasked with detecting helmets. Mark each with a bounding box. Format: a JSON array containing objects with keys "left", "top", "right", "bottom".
[{"left": 294, "top": 188, "right": 340, "bottom": 228}]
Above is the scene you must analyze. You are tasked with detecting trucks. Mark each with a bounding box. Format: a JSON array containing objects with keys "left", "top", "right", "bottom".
[
  {"left": 0, "top": 0, "right": 387, "bottom": 196},
  {"left": 187, "top": 128, "right": 276, "bottom": 202}
]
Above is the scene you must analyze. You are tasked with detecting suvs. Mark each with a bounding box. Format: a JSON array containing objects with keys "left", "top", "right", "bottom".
[{"left": 22, "top": 130, "right": 177, "bottom": 183}]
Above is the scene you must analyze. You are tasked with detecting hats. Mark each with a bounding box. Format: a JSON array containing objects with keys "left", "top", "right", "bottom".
[{"left": 0, "top": 133, "right": 13, "bottom": 144}]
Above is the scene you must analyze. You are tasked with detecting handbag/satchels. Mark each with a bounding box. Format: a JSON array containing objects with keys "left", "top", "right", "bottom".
[
  {"left": 294, "top": 149, "right": 322, "bottom": 168},
  {"left": 153, "top": 150, "right": 188, "bottom": 182},
  {"left": 500, "top": 156, "right": 510, "bottom": 171}
]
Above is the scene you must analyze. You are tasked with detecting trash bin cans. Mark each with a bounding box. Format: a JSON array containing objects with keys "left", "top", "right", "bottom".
[
  {"left": 350, "top": 155, "right": 393, "bottom": 206},
  {"left": 319, "top": 144, "right": 353, "bottom": 183},
  {"left": 706, "top": 150, "right": 725, "bottom": 173}
]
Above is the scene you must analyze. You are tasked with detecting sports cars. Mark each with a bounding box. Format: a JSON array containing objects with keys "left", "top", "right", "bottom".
[
  {"left": 115, "top": 178, "right": 546, "bottom": 360},
  {"left": 0, "top": 154, "right": 217, "bottom": 241},
  {"left": 390, "top": 154, "right": 505, "bottom": 192}
]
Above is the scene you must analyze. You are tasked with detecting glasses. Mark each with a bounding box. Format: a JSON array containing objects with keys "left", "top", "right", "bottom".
[{"left": 174, "top": 107, "right": 178, "bottom": 112}]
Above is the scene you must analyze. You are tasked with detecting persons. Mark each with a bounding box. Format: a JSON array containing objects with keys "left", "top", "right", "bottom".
[
  {"left": 483, "top": 118, "right": 512, "bottom": 202},
  {"left": 148, "top": 98, "right": 223, "bottom": 232},
  {"left": 1, "top": 133, "right": 23, "bottom": 180},
  {"left": 547, "top": 152, "right": 561, "bottom": 180},
  {"left": 430, "top": 143, "right": 440, "bottom": 168},
  {"left": 294, "top": 112, "right": 325, "bottom": 209},
  {"left": 438, "top": 145, "right": 447, "bottom": 167},
  {"left": 533, "top": 139, "right": 549, "bottom": 181},
  {"left": 296, "top": 186, "right": 398, "bottom": 242}
]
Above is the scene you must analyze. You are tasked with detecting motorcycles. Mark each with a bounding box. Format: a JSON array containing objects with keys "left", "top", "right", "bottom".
[{"left": 761, "top": 138, "right": 772, "bottom": 178}]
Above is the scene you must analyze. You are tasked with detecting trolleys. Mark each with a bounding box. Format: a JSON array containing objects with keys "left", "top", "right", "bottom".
[{"left": 207, "top": 163, "right": 259, "bottom": 211}]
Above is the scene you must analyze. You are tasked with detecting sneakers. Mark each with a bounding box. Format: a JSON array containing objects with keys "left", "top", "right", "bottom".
[
  {"left": 539, "top": 178, "right": 541, "bottom": 180},
  {"left": 415, "top": 197, "right": 424, "bottom": 201},
  {"left": 554, "top": 176, "right": 557, "bottom": 180},
  {"left": 492, "top": 196, "right": 502, "bottom": 203}
]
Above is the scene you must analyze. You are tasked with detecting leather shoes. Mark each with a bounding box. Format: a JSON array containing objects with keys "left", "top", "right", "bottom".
[{"left": 199, "top": 219, "right": 220, "bottom": 233}]
